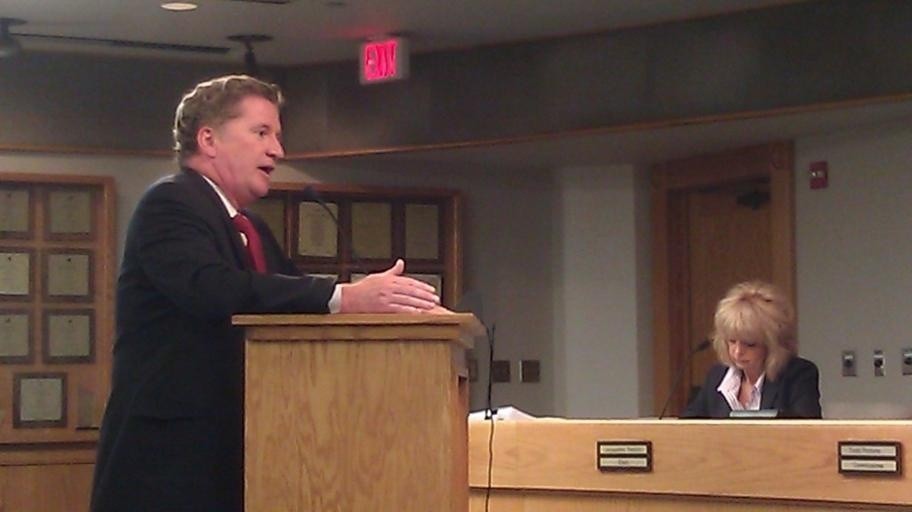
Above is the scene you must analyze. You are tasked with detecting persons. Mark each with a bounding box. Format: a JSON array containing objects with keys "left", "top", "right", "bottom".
[
  {"left": 674, "top": 277, "right": 823, "bottom": 419},
  {"left": 89, "top": 74, "right": 453, "bottom": 511}
]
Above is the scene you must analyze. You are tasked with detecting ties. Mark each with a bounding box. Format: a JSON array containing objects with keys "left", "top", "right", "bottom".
[{"left": 234, "top": 215, "right": 266, "bottom": 271}]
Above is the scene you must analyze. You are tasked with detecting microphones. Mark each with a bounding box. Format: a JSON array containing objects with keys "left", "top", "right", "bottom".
[
  {"left": 302, "top": 186, "right": 370, "bottom": 274},
  {"left": 659, "top": 340, "right": 710, "bottom": 419}
]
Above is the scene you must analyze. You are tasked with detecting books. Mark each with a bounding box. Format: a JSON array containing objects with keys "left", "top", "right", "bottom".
[{"left": 726, "top": 406, "right": 779, "bottom": 419}]
